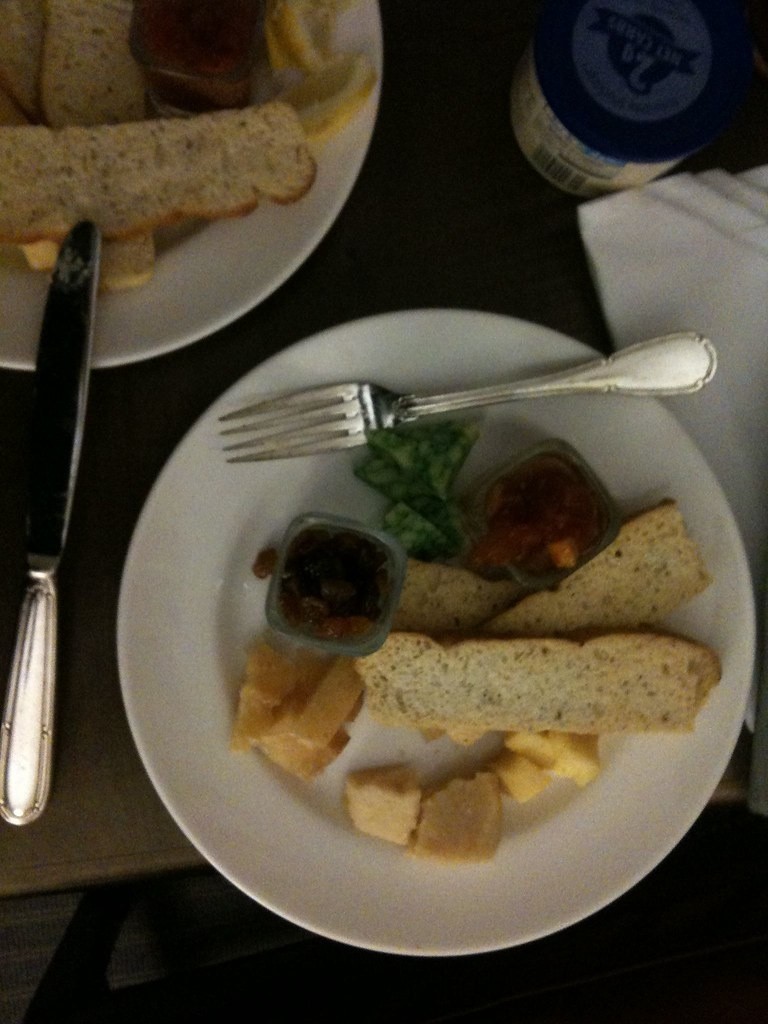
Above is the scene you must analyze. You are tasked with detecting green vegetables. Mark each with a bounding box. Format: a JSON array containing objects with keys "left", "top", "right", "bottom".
[{"left": 352, "top": 420, "right": 486, "bottom": 568}]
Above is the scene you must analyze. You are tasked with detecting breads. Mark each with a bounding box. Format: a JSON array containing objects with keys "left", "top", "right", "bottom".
[
  {"left": 0, "top": 0, "right": 370, "bottom": 290},
  {"left": 352, "top": 502, "right": 724, "bottom": 743}
]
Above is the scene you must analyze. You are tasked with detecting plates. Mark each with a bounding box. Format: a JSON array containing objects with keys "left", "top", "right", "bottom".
[
  {"left": 118, "top": 307, "right": 754, "bottom": 958},
  {"left": 0, "top": 0, "right": 382, "bottom": 372}
]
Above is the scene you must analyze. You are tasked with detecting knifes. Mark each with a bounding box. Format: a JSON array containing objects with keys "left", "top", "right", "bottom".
[{"left": 0, "top": 218, "right": 101, "bottom": 825}]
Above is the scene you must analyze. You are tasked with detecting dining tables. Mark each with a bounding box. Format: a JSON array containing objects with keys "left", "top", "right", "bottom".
[{"left": 0, "top": 0, "right": 768, "bottom": 895}]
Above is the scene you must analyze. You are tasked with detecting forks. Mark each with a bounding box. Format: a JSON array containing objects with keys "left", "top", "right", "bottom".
[{"left": 218, "top": 324, "right": 716, "bottom": 465}]
